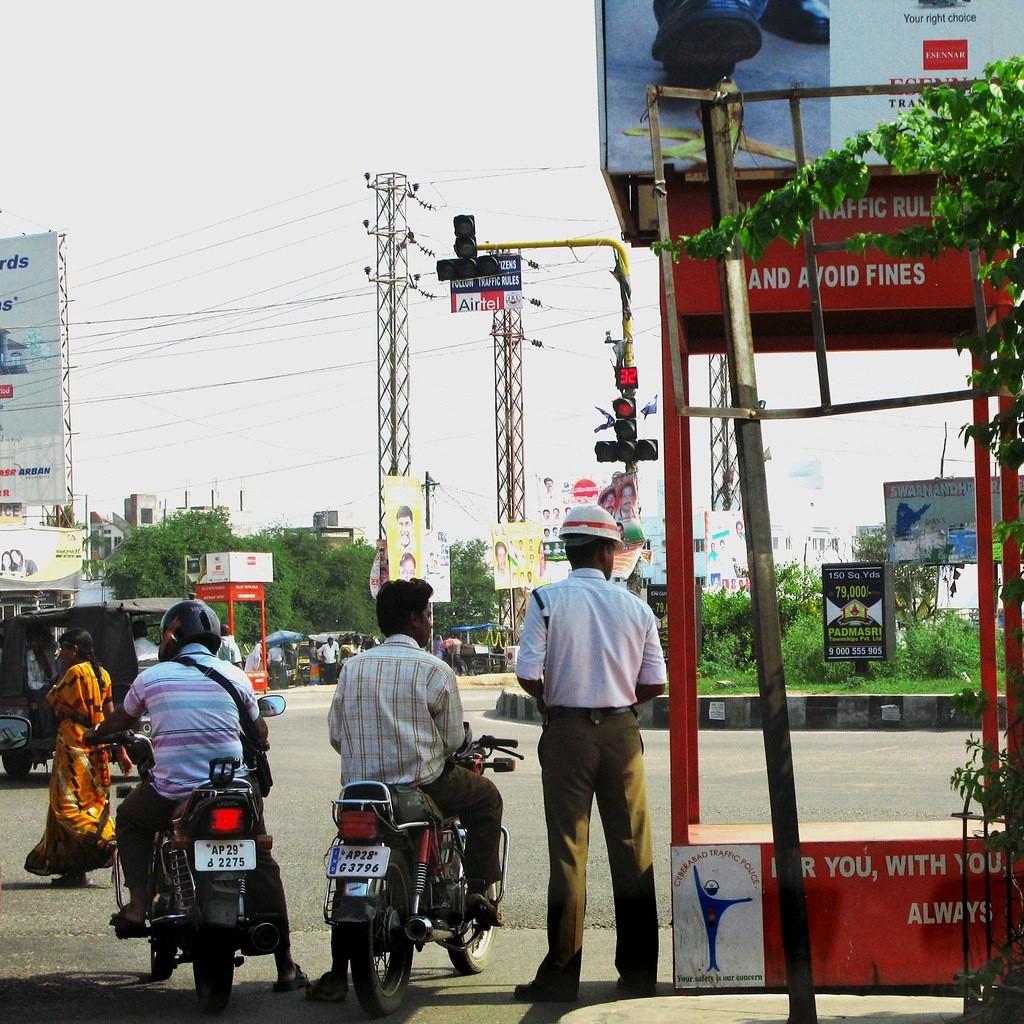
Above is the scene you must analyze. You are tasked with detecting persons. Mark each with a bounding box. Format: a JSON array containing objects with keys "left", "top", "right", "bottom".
[
  {"left": 132, "top": 618, "right": 510, "bottom": 691},
  {"left": 306, "top": 578, "right": 504, "bottom": 1002},
  {"left": 25, "top": 628, "right": 133, "bottom": 887},
  {"left": 510, "top": 503, "right": 667, "bottom": 1004},
  {"left": 82, "top": 601, "right": 312, "bottom": 994},
  {"left": 651, "top": 0, "right": 830, "bottom": 90}
]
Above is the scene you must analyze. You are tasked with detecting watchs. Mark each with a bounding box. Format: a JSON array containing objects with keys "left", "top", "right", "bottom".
[{"left": 94, "top": 723, "right": 107, "bottom": 737}]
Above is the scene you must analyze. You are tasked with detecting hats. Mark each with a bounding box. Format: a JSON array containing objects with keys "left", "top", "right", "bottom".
[{"left": 353, "top": 634, "right": 363, "bottom": 645}]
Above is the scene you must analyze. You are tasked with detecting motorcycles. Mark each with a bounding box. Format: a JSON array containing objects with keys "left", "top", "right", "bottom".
[
  {"left": 323, "top": 719, "right": 525, "bottom": 1022},
  {"left": 91, "top": 692, "right": 288, "bottom": 1017}
]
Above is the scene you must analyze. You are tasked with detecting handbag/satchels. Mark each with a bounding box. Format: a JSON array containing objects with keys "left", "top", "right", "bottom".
[{"left": 244, "top": 737, "right": 273, "bottom": 799}]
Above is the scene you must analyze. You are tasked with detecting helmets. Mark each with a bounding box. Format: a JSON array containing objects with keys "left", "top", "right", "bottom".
[
  {"left": 556, "top": 503, "right": 625, "bottom": 552},
  {"left": 158, "top": 599, "right": 222, "bottom": 662}
]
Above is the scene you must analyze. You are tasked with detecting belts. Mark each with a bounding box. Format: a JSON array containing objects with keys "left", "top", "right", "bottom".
[{"left": 547, "top": 704, "right": 633, "bottom": 715}]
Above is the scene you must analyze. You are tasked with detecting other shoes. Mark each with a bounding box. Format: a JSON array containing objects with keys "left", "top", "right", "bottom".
[
  {"left": 615, "top": 977, "right": 656, "bottom": 997},
  {"left": 305, "top": 972, "right": 348, "bottom": 1002},
  {"left": 652, "top": 2, "right": 762, "bottom": 95},
  {"left": 467, "top": 890, "right": 505, "bottom": 927},
  {"left": 513, "top": 979, "right": 577, "bottom": 1004},
  {"left": 756, "top": 1, "right": 829, "bottom": 45}
]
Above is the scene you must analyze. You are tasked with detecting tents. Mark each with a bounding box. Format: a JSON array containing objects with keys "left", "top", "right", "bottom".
[{"left": 451, "top": 623, "right": 510, "bottom": 644}]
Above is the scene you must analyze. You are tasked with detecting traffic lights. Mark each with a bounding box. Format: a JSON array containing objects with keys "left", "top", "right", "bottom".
[
  {"left": 617, "top": 366, "right": 638, "bottom": 390},
  {"left": 593, "top": 438, "right": 659, "bottom": 463},
  {"left": 454, "top": 213, "right": 477, "bottom": 259},
  {"left": 613, "top": 396, "right": 638, "bottom": 441},
  {"left": 436, "top": 255, "right": 504, "bottom": 282}
]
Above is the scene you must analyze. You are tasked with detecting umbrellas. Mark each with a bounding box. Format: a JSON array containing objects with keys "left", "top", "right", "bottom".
[{"left": 256, "top": 628, "right": 304, "bottom": 651}]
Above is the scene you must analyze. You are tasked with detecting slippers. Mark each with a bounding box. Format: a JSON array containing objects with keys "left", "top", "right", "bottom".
[
  {"left": 271, "top": 963, "right": 309, "bottom": 993},
  {"left": 48, "top": 874, "right": 95, "bottom": 889},
  {"left": 108, "top": 903, "right": 144, "bottom": 933},
  {"left": 97, "top": 845, "right": 115, "bottom": 868}
]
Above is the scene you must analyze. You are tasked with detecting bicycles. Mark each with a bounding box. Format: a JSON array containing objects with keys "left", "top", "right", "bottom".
[{"left": 442, "top": 650, "right": 489, "bottom": 677}]
